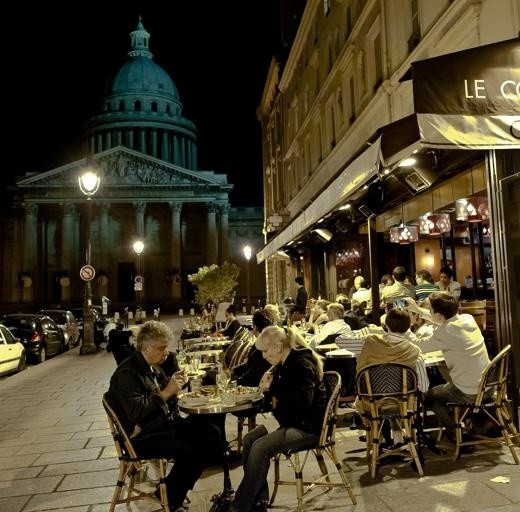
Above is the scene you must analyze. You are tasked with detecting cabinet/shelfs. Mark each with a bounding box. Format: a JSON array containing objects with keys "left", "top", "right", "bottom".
[
  {"left": 28, "top": 367, "right": 31, "bottom": 372},
  {"left": 477, "top": 279, "right": 483, "bottom": 287},
  {"left": 318, "top": 296, "right": 321, "bottom": 300}
]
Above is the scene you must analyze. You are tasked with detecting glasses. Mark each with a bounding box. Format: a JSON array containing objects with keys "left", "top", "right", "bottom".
[
  {"left": 456, "top": 166, "right": 490, "bottom": 222},
  {"left": 419, "top": 186, "right": 452, "bottom": 235},
  {"left": 390, "top": 197, "right": 419, "bottom": 245}
]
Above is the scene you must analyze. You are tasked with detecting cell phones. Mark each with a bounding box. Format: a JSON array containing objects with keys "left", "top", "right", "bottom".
[{"left": 396, "top": 299, "right": 406, "bottom": 306}]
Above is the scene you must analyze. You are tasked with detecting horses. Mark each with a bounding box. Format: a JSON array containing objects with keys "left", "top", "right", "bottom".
[
  {"left": 182, "top": 366, "right": 188, "bottom": 375},
  {"left": 234, "top": 399, "right": 252, "bottom": 406}
]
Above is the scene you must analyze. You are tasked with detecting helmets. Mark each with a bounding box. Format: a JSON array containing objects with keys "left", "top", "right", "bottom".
[{"left": 178, "top": 387, "right": 256, "bottom": 405}]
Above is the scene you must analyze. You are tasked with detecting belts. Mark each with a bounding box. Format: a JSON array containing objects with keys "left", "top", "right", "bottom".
[
  {"left": 215, "top": 372, "right": 231, "bottom": 400},
  {"left": 177, "top": 351, "right": 201, "bottom": 373}
]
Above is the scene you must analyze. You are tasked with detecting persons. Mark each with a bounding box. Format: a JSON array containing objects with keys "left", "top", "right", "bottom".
[
  {"left": 202, "top": 266, "right": 460, "bottom": 345},
  {"left": 186, "top": 310, "right": 278, "bottom": 422},
  {"left": 109, "top": 320, "right": 221, "bottom": 512},
  {"left": 393, "top": 291, "right": 502, "bottom": 453},
  {"left": 221, "top": 325, "right": 334, "bottom": 512},
  {"left": 334, "top": 307, "right": 430, "bottom": 446}
]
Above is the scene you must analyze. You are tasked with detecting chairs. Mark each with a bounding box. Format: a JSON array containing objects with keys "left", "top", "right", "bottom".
[
  {"left": 103, "top": 392, "right": 190, "bottom": 512},
  {"left": 356, "top": 362, "right": 424, "bottom": 480},
  {"left": 269, "top": 370, "right": 357, "bottom": 512},
  {"left": 446, "top": 344, "right": 520, "bottom": 465},
  {"left": 101, "top": 307, "right": 490, "bottom": 397}
]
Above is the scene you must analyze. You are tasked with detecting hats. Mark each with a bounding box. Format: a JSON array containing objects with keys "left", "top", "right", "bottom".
[
  {"left": 0, "top": 323, "right": 27, "bottom": 378},
  {"left": 38, "top": 309, "right": 80, "bottom": 351},
  {"left": 69, "top": 307, "right": 100, "bottom": 341},
  {"left": 87, "top": 304, "right": 109, "bottom": 327},
  {"left": 0, "top": 312, "right": 65, "bottom": 364}
]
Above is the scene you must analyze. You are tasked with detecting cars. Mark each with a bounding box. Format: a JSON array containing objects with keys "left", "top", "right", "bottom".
[{"left": 396, "top": 299, "right": 405, "bottom": 307}]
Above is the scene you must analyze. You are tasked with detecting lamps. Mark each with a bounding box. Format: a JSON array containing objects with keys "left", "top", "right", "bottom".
[
  {"left": 419, "top": 186, "right": 452, "bottom": 235},
  {"left": 456, "top": 166, "right": 490, "bottom": 222},
  {"left": 390, "top": 197, "right": 419, "bottom": 245}
]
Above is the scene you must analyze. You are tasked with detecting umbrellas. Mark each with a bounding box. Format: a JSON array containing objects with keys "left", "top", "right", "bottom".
[{"left": 155, "top": 489, "right": 190, "bottom": 512}]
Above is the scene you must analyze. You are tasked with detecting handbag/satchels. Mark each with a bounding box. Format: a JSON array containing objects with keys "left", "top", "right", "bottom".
[{"left": 160, "top": 391, "right": 168, "bottom": 400}]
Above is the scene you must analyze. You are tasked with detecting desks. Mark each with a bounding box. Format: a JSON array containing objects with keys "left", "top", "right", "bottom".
[
  {"left": 174, "top": 386, "right": 264, "bottom": 512},
  {"left": 78, "top": 153, "right": 101, "bottom": 355},
  {"left": 133, "top": 230, "right": 145, "bottom": 325},
  {"left": 243, "top": 243, "right": 252, "bottom": 315}
]
